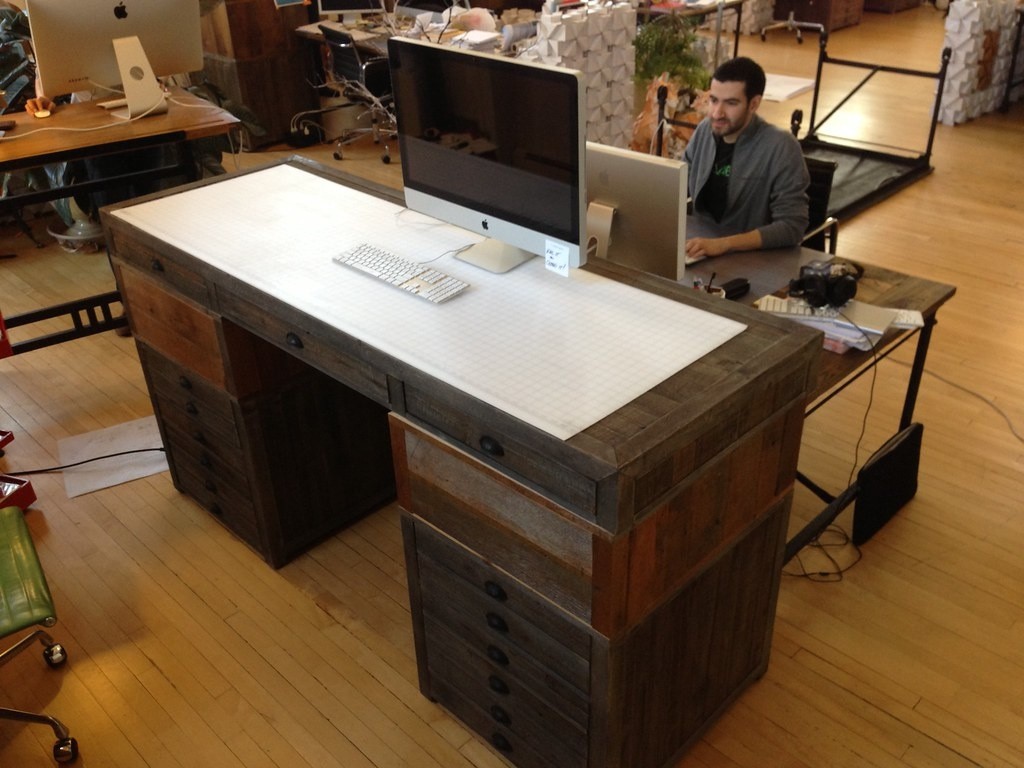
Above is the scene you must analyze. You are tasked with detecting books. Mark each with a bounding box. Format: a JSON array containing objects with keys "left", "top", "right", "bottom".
[{"left": 752, "top": 285, "right": 897, "bottom": 353}]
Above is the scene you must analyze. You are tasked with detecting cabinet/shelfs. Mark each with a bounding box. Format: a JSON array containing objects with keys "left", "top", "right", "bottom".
[{"left": 96, "top": 156, "right": 823, "bottom": 768}]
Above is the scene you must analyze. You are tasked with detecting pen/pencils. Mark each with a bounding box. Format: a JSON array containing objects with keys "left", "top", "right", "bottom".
[{"left": 706, "top": 272, "right": 717, "bottom": 292}]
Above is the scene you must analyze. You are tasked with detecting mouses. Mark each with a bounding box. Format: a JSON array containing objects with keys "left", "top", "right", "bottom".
[{"left": 33, "top": 109, "right": 51, "bottom": 118}]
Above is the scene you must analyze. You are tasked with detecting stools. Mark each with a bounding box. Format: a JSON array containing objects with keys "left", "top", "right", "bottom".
[{"left": 0, "top": 504, "right": 78, "bottom": 764}]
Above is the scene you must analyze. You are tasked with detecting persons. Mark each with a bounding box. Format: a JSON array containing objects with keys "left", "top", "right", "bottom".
[
  {"left": 674, "top": 57, "right": 810, "bottom": 260},
  {"left": 24, "top": 67, "right": 169, "bottom": 118}
]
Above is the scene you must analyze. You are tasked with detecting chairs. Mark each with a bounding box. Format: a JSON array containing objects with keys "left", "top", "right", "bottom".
[
  {"left": 317, "top": 24, "right": 399, "bottom": 164},
  {"left": 798, "top": 157, "right": 838, "bottom": 255}
]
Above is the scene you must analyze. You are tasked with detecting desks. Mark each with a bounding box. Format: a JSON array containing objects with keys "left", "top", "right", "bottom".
[
  {"left": 0, "top": 87, "right": 239, "bottom": 357},
  {"left": 688, "top": 214, "right": 958, "bottom": 563},
  {"left": 294, "top": 8, "right": 532, "bottom": 60}
]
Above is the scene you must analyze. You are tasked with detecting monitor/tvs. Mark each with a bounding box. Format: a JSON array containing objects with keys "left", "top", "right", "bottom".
[
  {"left": 385, "top": 36, "right": 587, "bottom": 276},
  {"left": 585, "top": 142, "right": 688, "bottom": 283},
  {"left": 317, "top": 0, "right": 385, "bottom": 28},
  {"left": 25, "top": 0, "right": 205, "bottom": 119}
]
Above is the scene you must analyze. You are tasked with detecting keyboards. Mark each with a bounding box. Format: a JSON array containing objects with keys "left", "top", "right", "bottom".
[
  {"left": 97, "top": 91, "right": 171, "bottom": 110},
  {"left": 333, "top": 241, "right": 470, "bottom": 307},
  {"left": 685, "top": 251, "right": 707, "bottom": 268}
]
